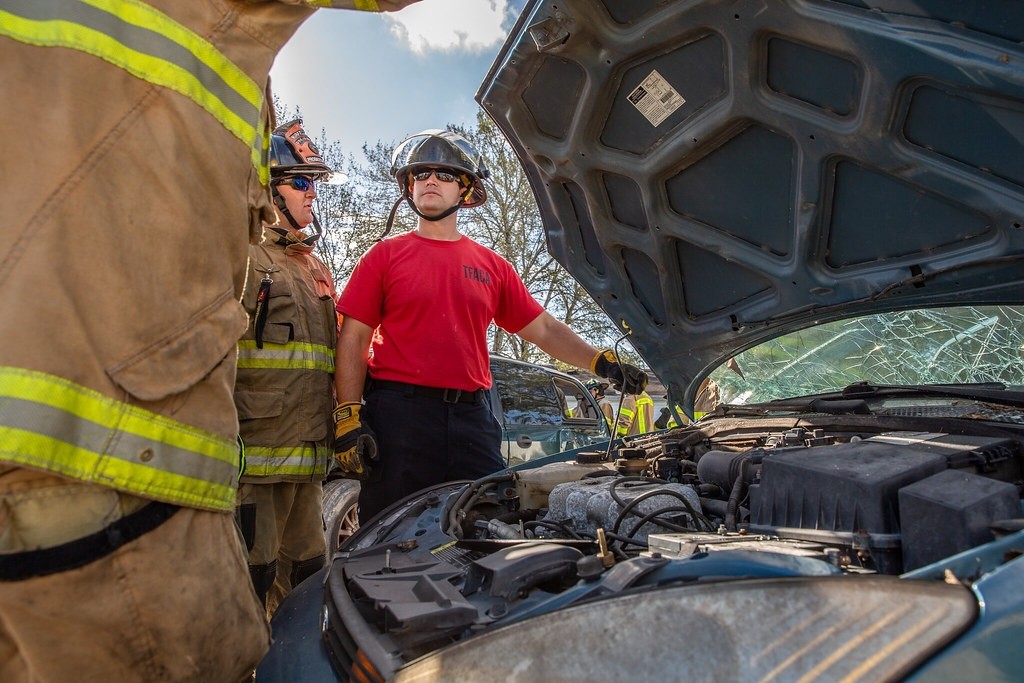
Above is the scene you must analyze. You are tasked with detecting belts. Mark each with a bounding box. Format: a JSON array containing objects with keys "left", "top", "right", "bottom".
[{"left": 371, "top": 378, "right": 483, "bottom": 405}]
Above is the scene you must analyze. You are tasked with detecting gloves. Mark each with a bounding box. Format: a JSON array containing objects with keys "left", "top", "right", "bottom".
[
  {"left": 590, "top": 349, "right": 648, "bottom": 395},
  {"left": 331, "top": 401, "right": 378, "bottom": 474}
]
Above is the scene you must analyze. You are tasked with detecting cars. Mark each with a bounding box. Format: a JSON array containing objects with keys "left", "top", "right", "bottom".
[
  {"left": 250, "top": 0, "right": 1024, "bottom": 683},
  {"left": 321, "top": 349, "right": 612, "bottom": 569}
]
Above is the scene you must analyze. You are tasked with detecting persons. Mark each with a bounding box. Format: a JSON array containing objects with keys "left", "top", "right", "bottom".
[
  {"left": 1, "top": 1, "right": 419, "bottom": 683},
  {"left": 557, "top": 378, "right": 722, "bottom": 446},
  {"left": 232, "top": 118, "right": 339, "bottom": 612},
  {"left": 332, "top": 128, "right": 650, "bottom": 527}
]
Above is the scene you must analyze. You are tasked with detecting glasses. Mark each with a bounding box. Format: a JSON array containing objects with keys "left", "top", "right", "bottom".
[
  {"left": 412, "top": 167, "right": 463, "bottom": 189},
  {"left": 277, "top": 177, "right": 313, "bottom": 192}
]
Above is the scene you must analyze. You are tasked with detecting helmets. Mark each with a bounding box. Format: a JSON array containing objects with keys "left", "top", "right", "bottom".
[
  {"left": 585, "top": 379, "right": 609, "bottom": 392},
  {"left": 390, "top": 130, "right": 486, "bottom": 207},
  {"left": 270, "top": 134, "right": 333, "bottom": 181}
]
